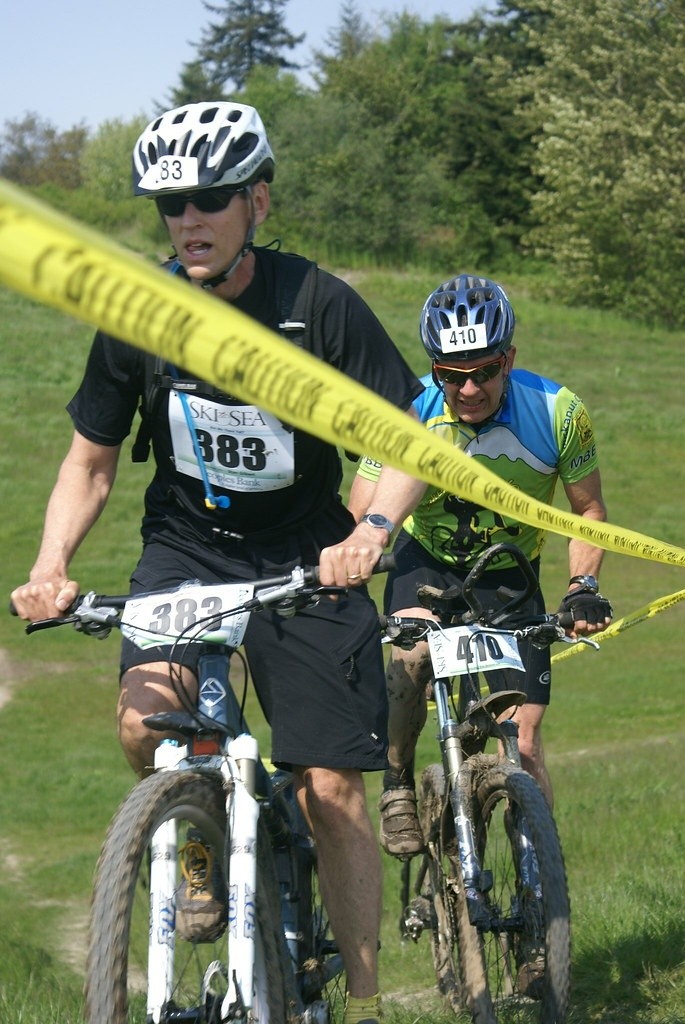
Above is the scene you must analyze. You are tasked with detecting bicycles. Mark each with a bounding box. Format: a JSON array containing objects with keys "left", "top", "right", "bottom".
[
  {"left": 376, "top": 541, "right": 610, "bottom": 1024},
  {"left": 8, "top": 548, "right": 399, "bottom": 1024}
]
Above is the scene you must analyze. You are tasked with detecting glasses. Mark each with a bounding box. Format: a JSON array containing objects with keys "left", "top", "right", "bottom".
[
  {"left": 433, "top": 353, "right": 508, "bottom": 386},
  {"left": 154, "top": 188, "right": 246, "bottom": 217}
]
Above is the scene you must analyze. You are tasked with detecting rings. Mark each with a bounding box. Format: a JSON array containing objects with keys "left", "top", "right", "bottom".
[{"left": 346, "top": 573, "right": 360, "bottom": 580}]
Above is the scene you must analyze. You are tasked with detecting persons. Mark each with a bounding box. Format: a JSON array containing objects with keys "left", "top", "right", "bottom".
[
  {"left": 347, "top": 273, "right": 615, "bottom": 1002},
  {"left": 10, "top": 102, "right": 429, "bottom": 1024}
]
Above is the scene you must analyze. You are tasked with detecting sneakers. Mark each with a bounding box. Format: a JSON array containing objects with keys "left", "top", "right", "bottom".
[
  {"left": 379, "top": 787, "right": 422, "bottom": 856},
  {"left": 509, "top": 919, "right": 543, "bottom": 1001},
  {"left": 172, "top": 838, "right": 228, "bottom": 942},
  {"left": 357, "top": 1018, "right": 379, "bottom": 1024}
]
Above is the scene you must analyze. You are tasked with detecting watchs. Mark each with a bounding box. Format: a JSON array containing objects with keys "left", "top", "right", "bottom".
[
  {"left": 360, "top": 513, "right": 396, "bottom": 548},
  {"left": 569, "top": 573, "right": 599, "bottom": 594}
]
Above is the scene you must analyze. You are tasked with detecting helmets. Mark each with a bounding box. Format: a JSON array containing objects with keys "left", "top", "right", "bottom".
[
  {"left": 418, "top": 273, "right": 516, "bottom": 360},
  {"left": 132, "top": 102, "right": 276, "bottom": 198}
]
